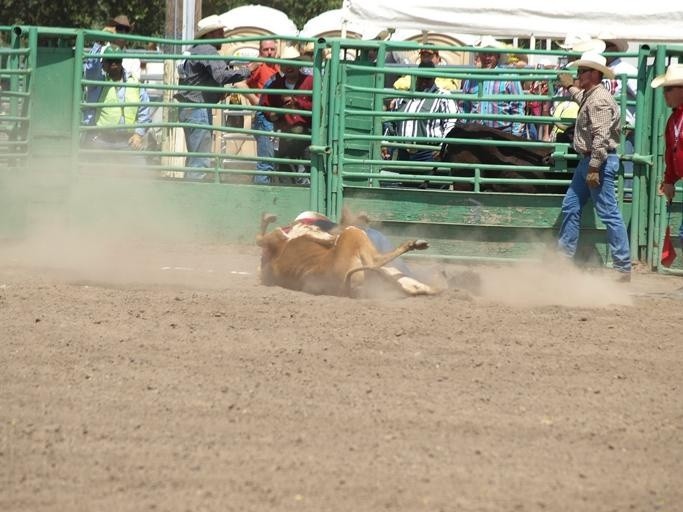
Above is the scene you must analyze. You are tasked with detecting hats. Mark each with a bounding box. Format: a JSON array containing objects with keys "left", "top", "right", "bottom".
[
  {"left": 103, "top": 46, "right": 121, "bottom": 53},
  {"left": 566, "top": 53, "right": 614, "bottom": 80},
  {"left": 277, "top": 47, "right": 310, "bottom": 61},
  {"left": 420, "top": 43, "right": 437, "bottom": 54},
  {"left": 110, "top": 15, "right": 134, "bottom": 30},
  {"left": 194, "top": 15, "right": 225, "bottom": 39},
  {"left": 473, "top": 35, "right": 507, "bottom": 65},
  {"left": 651, "top": 64, "right": 683, "bottom": 89}
]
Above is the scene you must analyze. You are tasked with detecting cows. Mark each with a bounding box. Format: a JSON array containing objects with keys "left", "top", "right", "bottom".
[
  {"left": 253, "top": 208, "right": 443, "bottom": 298},
  {"left": 416, "top": 120, "right": 580, "bottom": 196}
]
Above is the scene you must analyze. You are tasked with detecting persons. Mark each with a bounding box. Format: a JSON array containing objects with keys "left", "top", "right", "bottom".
[
  {"left": 83, "top": 14, "right": 162, "bottom": 164},
  {"left": 281, "top": 211, "right": 416, "bottom": 288},
  {"left": 233, "top": 38, "right": 330, "bottom": 187},
  {"left": 173, "top": 15, "right": 263, "bottom": 182},
  {"left": 359, "top": 26, "right": 638, "bottom": 199},
  {"left": 651, "top": 64, "right": 683, "bottom": 241},
  {"left": 547, "top": 51, "right": 632, "bottom": 284}
]
